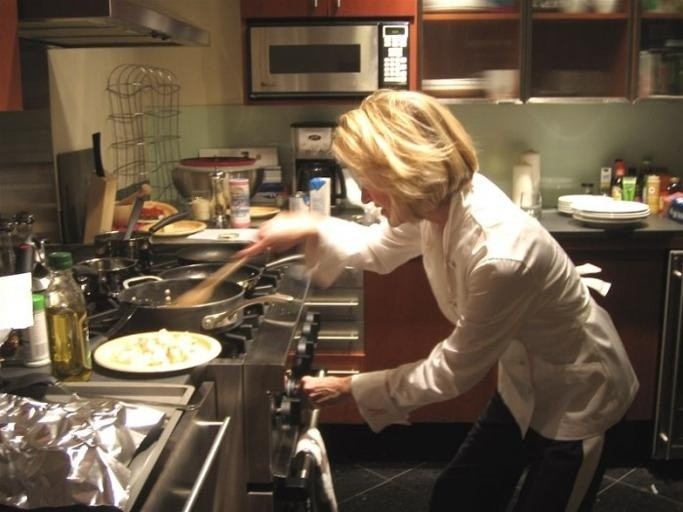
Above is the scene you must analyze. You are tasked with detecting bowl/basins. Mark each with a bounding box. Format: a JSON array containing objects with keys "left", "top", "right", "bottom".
[
  {"left": 482, "top": 68, "right": 519, "bottom": 101},
  {"left": 172, "top": 158, "right": 263, "bottom": 204}
]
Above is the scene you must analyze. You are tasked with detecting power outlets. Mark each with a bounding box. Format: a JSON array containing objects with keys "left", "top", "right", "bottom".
[{"left": 239, "top": 149, "right": 279, "bottom": 167}]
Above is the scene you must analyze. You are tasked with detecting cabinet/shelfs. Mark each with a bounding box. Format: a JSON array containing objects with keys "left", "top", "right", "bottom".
[
  {"left": 241, "top": 0, "right": 683, "bottom": 105},
  {"left": 313, "top": 209, "right": 683, "bottom": 422}
]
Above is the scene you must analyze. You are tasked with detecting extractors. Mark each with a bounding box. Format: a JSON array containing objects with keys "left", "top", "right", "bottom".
[{"left": 15, "top": 0, "right": 210, "bottom": 50}]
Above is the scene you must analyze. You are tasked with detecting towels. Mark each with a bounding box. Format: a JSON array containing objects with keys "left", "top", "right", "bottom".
[{"left": 296, "top": 428, "right": 339, "bottom": 512}]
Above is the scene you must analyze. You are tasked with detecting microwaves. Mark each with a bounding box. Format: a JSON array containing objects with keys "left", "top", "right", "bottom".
[{"left": 249, "top": 21, "right": 410, "bottom": 100}]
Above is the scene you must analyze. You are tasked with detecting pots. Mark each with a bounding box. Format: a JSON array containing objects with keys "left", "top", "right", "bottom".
[
  {"left": 161, "top": 252, "right": 313, "bottom": 296},
  {"left": 116, "top": 277, "right": 292, "bottom": 339},
  {"left": 175, "top": 245, "right": 270, "bottom": 269},
  {"left": 74, "top": 257, "right": 151, "bottom": 298},
  {"left": 94, "top": 211, "right": 191, "bottom": 263}
]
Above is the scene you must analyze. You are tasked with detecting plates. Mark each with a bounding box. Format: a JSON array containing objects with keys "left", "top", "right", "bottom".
[
  {"left": 227, "top": 206, "right": 280, "bottom": 219},
  {"left": 558, "top": 194, "right": 619, "bottom": 215},
  {"left": 541, "top": 69, "right": 615, "bottom": 97},
  {"left": 139, "top": 219, "right": 208, "bottom": 239},
  {"left": 421, "top": 79, "right": 487, "bottom": 97},
  {"left": 423, "top": 1, "right": 503, "bottom": 14},
  {"left": 93, "top": 330, "right": 223, "bottom": 376},
  {"left": 572, "top": 205, "right": 651, "bottom": 224}
]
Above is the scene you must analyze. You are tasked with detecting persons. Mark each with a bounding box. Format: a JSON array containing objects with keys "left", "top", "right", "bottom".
[{"left": 229, "top": 89, "right": 642, "bottom": 511}]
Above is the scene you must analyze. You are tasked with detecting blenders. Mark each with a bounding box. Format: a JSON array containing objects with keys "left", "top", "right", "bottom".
[{"left": 289, "top": 120, "right": 348, "bottom": 212}]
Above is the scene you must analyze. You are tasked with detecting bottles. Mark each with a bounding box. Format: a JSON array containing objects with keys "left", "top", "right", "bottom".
[
  {"left": 581, "top": 181, "right": 594, "bottom": 195},
  {"left": 638, "top": 161, "right": 652, "bottom": 205},
  {"left": 513, "top": 162, "right": 532, "bottom": 209},
  {"left": 522, "top": 149, "right": 540, "bottom": 191},
  {"left": 667, "top": 177, "right": 680, "bottom": 216},
  {"left": 230, "top": 179, "right": 250, "bottom": 229},
  {"left": 644, "top": 175, "right": 661, "bottom": 215},
  {"left": 611, "top": 159, "right": 626, "bottom": 200},
  {"left": 19, "top": 295, "right": 50, "bottom": 368},
  {"left": 44, "top": 253, "right": 92, "bottom": 384}
]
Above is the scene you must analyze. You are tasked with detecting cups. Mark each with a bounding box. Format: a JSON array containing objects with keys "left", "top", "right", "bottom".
[{"left": 521, "top": 191, "right": 543, "bottom": 219}]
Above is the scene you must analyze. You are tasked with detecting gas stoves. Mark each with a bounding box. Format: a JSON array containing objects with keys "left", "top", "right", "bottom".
[{"left": 39, "top": 258, "right": 321, "bottom": 489}]
